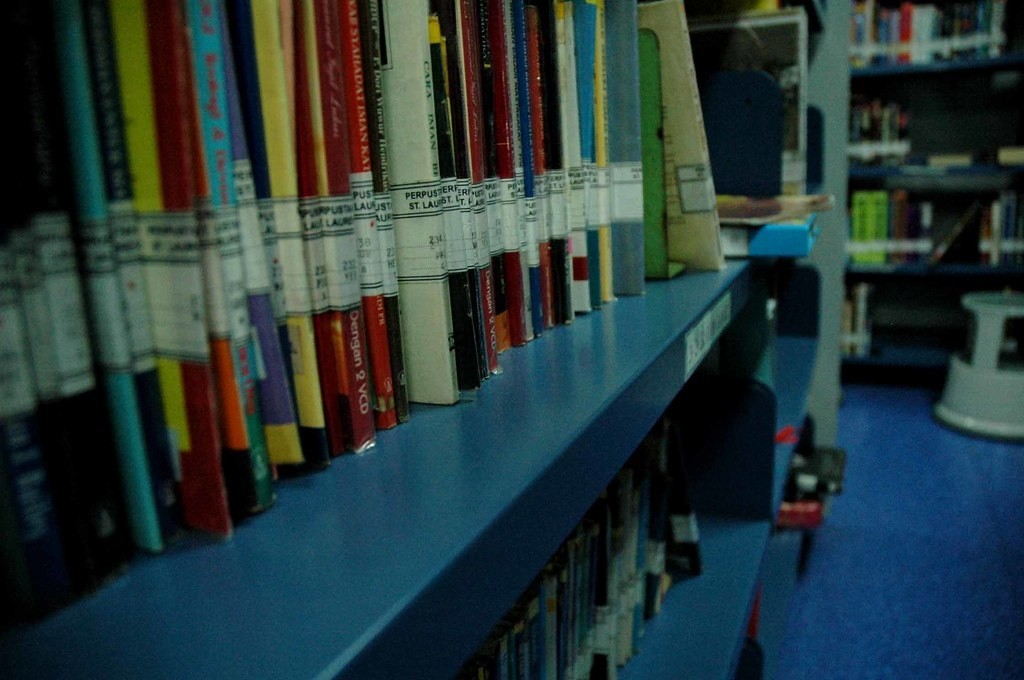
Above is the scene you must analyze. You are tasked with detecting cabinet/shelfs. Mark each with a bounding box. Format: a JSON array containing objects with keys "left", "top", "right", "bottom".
[{"left": 0, "top": 0, "right": 1024, "bottom": 680}]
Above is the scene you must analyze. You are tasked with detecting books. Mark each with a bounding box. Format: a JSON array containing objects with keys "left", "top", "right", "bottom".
[
  {"left": 638, "top": 0, "right": 724, "bottom": 274},
  {"left": 464, "top": 458, "right": 701, "bottom": 679},
  {"left": 842, "top": 0, "right": 1024, "bottom": 359},
  {"left": 0, "top": 0, "right": 647, "bottom": 630},
  {"left": 715, "top": 195, "right": 834, "bottom": 257}
]
